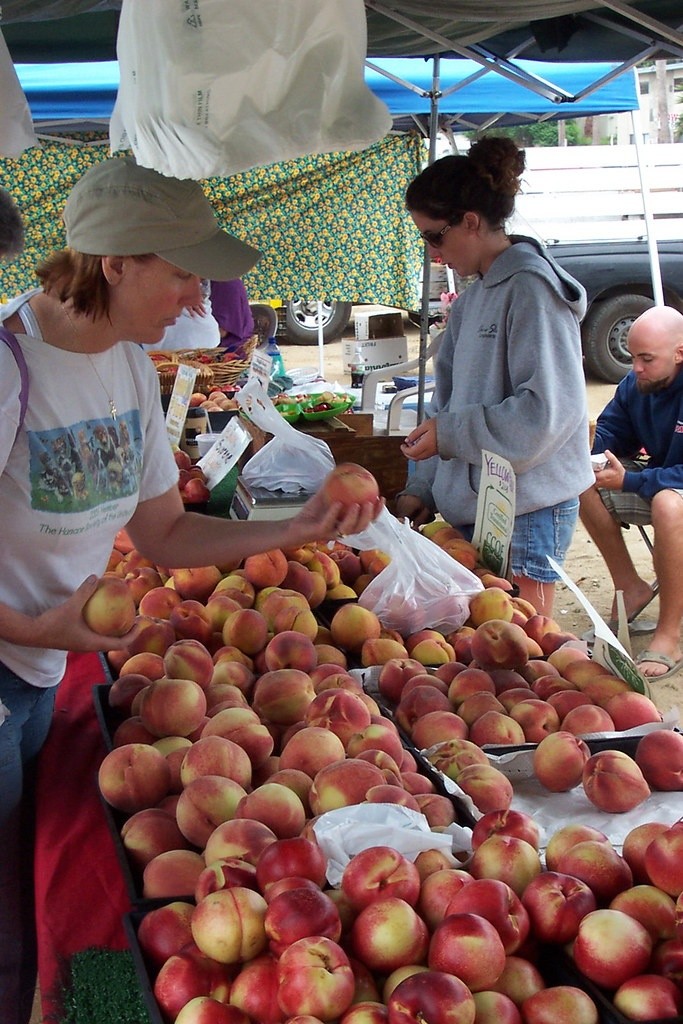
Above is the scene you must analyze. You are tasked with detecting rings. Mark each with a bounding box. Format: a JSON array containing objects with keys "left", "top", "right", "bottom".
[{"left": 337, "top": 531, "right": 345, "bottom": 538}]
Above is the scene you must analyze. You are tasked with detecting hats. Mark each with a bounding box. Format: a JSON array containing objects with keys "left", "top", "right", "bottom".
[{"left": 61, "top": 156, "right": 264, "bottom": 282}]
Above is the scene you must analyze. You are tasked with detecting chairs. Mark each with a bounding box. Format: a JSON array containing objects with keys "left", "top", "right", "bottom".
[{"left": 356, "top": 330, "right": 446, "bottom": 435}]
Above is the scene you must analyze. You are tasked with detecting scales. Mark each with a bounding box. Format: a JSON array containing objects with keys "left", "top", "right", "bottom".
[{"left": 226, "top": 464, "right": 338, "bottom": 524}]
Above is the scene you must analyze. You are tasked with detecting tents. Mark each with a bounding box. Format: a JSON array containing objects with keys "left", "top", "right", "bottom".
[{"left": 0, "top": 0, "right": 683, "bottom": 431}]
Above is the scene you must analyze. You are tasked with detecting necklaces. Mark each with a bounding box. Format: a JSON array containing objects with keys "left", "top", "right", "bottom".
[{"left": 61, "top": 305, "right": 118, "bottom": 423}]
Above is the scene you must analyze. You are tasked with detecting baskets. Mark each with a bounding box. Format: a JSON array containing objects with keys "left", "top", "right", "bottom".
[{"left": 151, "top": 334, "right": 259, "bottom": 396}]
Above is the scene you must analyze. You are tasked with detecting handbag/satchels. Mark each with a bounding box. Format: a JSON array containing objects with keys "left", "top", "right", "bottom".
[
  {"left": 235, "top": 378, "right": 337, "bottom": 493},
  {"left": 337, "top": 504, "right": 486, "bottom": 637}
]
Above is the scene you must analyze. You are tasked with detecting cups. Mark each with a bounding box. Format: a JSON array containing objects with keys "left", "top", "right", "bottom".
[{"left": 180, "top": 407, "right": 206, "bottom": 459}]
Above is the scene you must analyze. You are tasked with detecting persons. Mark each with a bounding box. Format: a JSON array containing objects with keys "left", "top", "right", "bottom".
[
  {"left": 394, "top": 136, "right": 589, "bottom": 623},
  {"left": 0, "top": 189, "right": 24, "bottom": 263},
  {"left": 579, "top": 306, "right": 683, "bottom": 682},
  {"left": 0, "top": 153, "right": 387, "bottom": 1024},
  {"left": 205, "top": 275, "right": 254, "bottom": 348},
  {"left": 140, "top": 277, "right": 221, "bottom": 353}
]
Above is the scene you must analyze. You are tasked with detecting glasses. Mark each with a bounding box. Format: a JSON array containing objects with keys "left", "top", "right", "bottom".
[{"left": 420, "top": 214, "right": 461, "bottom": 248}]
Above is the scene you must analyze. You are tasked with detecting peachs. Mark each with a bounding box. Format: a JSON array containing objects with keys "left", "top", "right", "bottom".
[
  {"left": 189, "top": 390, "right": 237, "bottom": 412},
  {"left": 269, "top": 391, "right": 349, "bottom": 415},
  {"left": 323, "top": 462, "right": 379, "bottom": 520},
  {"left": 82, "top": 521, "right": 683, "bottom": 1024},
  {"left": 167, "top": 352, "right": 239, "bottom": 375},
  {"left": 174, "top": 449, "right": 211, "bottom": 503}
]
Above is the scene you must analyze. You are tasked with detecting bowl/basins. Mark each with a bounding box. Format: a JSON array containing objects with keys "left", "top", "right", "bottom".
[
  {"left": 591, "top": 453, "right": 608, "bottom": 471},
  {"left": 195, "top": 434, "right": 221, "bottom": 457},
  {"left": 285, "top": 367, "right": 319, "bottom": 384},
  {"left": 207, "top": 410, "right": 239, "bottom": 434},
  {"left": 221, "top": 390, "right": 236, "bottom": 401}
]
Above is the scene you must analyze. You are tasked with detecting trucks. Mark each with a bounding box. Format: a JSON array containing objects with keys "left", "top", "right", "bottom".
[{"left": 248, "top": 132, "right": 683, "bottom": 384}]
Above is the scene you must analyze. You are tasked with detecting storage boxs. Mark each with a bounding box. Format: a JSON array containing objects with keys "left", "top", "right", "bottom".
[
  {"left": 353, "top": 309, "right": 405, "bottom": 339},
  {"left": 340, "top": 336, "right": 409, "bottom": 376},
  {"left": 237, "top": 408, "right": 409, "bottom": 502}
]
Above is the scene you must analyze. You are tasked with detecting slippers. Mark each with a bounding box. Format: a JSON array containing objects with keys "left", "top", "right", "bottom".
[
  {"left": 582, "top": 620, "right": 658, "bottom": 646},
  {"left": 633, "top": 649, "right": 683, "bottom": 683}
]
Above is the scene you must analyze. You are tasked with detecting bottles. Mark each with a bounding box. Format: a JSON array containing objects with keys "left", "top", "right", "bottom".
[
  {"left": 351, "top": 347, "right": 365, "bottom": 388},
  {"left": 264, "top": 337, "right": 281, "bottom": 357}
]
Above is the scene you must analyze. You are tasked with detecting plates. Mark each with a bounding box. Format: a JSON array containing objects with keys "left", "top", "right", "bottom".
[{"left": 238, "top": 392, "right": 356, "bottom": 423}]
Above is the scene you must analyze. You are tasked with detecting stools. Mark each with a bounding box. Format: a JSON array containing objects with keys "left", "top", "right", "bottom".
[{"left": 629, "top": 520, "right": 660, "bottom": 628}]
